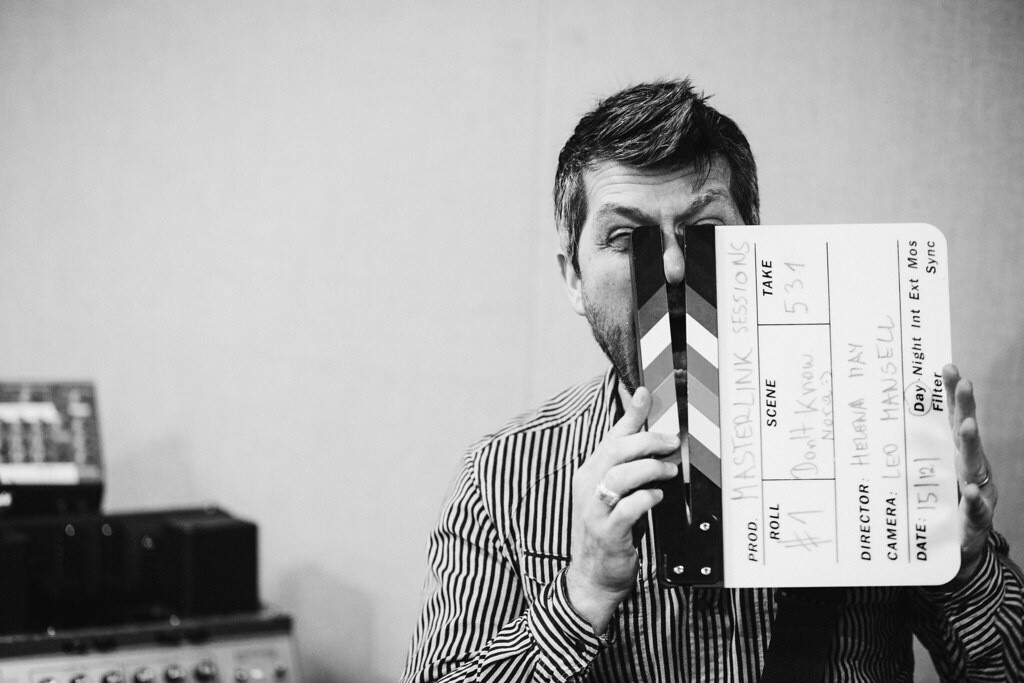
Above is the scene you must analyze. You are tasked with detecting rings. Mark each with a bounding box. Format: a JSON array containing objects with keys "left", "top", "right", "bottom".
[{"left": 975, "top": 471, "right": 990, "bottom": 488}]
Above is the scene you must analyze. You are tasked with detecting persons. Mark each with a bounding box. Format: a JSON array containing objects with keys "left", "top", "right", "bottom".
[{"left": 400, "top": 80, "right": 1024, "bottom": 683}]
